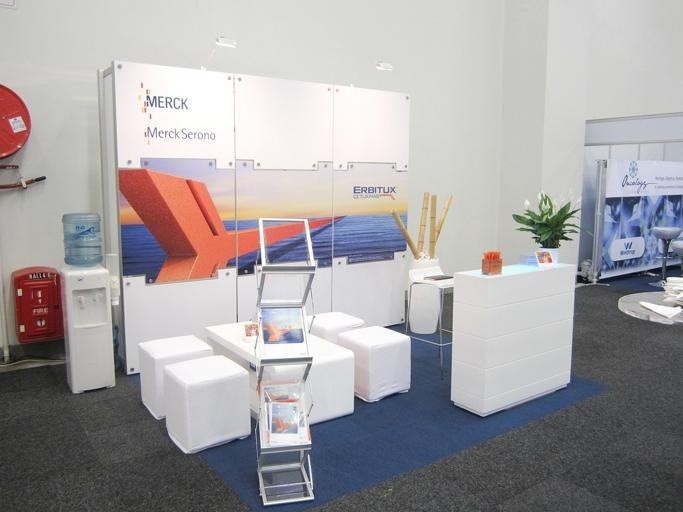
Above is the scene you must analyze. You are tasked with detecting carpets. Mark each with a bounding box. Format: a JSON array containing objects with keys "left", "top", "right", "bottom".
[{"left": 159, "top": 324, "right": 609, "bottom": 511}]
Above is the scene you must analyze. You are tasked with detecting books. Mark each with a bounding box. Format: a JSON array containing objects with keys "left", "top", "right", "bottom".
[
  {"left": 257, "top": 381, "right": 311, "bottom": 449},
  {"left": 258, "top": 308, "right": 309, "bottom": 362}
]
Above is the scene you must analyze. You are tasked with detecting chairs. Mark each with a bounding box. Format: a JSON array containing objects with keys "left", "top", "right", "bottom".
[{"left": 404, "top": 282, "right": 454, "bottom": 380}]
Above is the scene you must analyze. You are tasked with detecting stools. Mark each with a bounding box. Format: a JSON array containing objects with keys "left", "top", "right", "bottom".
[
  {"left": 139, "top": 310, "right": 411, "bottom": 457},
  {"left": 649, "top": 226, "right": 683, "bottom": 286}
]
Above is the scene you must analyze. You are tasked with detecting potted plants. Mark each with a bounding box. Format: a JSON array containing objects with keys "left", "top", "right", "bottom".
[{"left": 512, "top": 190, "right": 585, "bottom": 265}]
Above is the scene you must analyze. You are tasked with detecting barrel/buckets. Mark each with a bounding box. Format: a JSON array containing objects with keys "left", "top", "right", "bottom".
[{"left": 62, "top": 213, "right": 103, "bottom": 268}]
